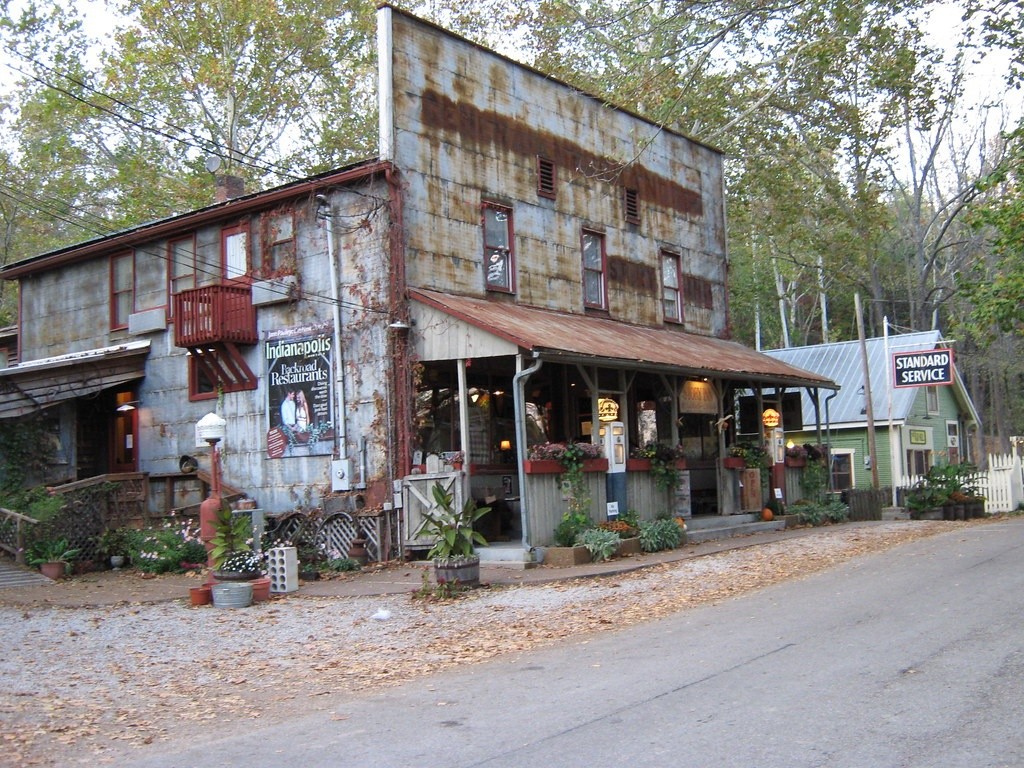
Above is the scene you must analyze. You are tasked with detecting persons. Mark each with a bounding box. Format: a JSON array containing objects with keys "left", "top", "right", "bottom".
[{"left": 280, "top": 383, "right": 309, "bottom": 427}]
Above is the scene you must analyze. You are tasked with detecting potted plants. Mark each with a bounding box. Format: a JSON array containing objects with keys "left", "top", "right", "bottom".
[
  {"left": 904, "top": 457, "right": 989, "bottom": 522},
  {"left": 28, "top": 538, "right": 82, "bottom": 580},
  {"left": 415, "top": 479, "right": 493, "bottom": 586}
]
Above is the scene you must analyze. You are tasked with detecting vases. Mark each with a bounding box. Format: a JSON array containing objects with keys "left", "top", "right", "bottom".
[
  {"left": 784, "top": 456, "right": 806, "bottom": 467},
  {"left": 190, "top": 581, "right": 215, "bottom": 605},
  {"left": 453, "top": 463, "right": 475, "bottom": 476},
  {"left": 724, "top": 456, "right": 745, "bottom": 469},
  {"left": 99, "top": 526, "right": 124, "bottom": 571},
  {"left": 246, "top": 579, "right": 270, "bottom": 601},
  {"left": 524, "top": 458, "right": 609, "bottom": 474},
  {"left": 211, "top": 570, "right": 261, "bottom": 583},
  {"left": 625, "top": 456, "right": 687, "bottom": 471}
]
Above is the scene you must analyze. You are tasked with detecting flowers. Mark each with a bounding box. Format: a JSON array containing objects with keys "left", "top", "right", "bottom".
[
  {"left": 446, "top": 451, "right": 464, "bottom": 465},
  {"left": 631, "top": 444, "right": 685, "bottom": 492},
  {"left": 526, "top": 443, "right": 601, "bottom": 461},
  {"left": 786, "top": 445, "right": 808, "bottom": 458},
  {"left": 204, "top": 497, "right": 264, "bottom": 574},
  {"left": 729, "top": 446, "right": 748, "bottom": 458}
]
